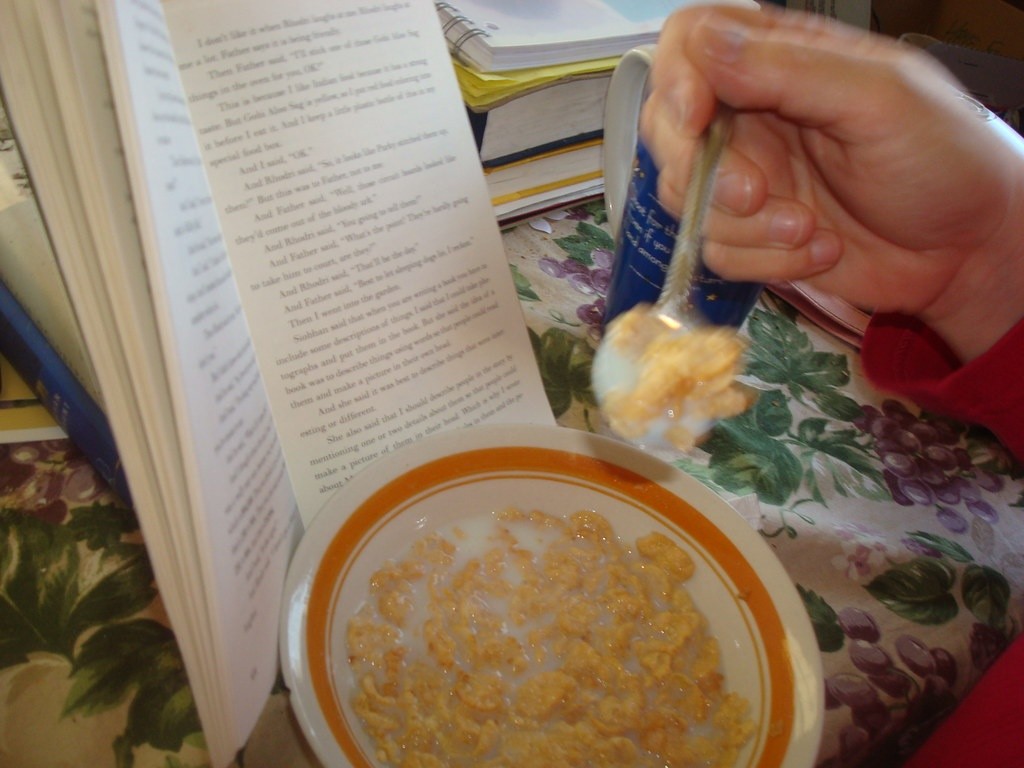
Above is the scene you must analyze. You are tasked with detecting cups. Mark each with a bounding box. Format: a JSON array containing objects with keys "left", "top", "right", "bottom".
[{"left": 603, "top": 44, "right": 767, "bottom": 336}]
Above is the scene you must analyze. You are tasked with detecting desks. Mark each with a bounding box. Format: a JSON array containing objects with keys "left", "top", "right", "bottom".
[{"left": 0, "top": 197, "right": 1024, "bottom": 768}]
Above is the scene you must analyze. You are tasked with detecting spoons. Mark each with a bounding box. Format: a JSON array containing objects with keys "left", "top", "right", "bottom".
[{"left": 591, "top": 103, "right": 736, "bottom": 447}]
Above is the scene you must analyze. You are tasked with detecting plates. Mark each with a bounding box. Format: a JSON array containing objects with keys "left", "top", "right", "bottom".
[{"left": 281, "top": 424, "right": 825, "bottom": 768}]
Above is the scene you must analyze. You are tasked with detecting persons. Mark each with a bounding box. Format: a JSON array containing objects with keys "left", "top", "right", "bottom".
[{"left": 640, "top": 8, "right": 1024, "bottom": 768}]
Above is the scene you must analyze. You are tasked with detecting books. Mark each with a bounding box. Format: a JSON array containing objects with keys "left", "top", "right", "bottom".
[{"left": 0, "top": 0, "right": 762, "bottom": 767}]
